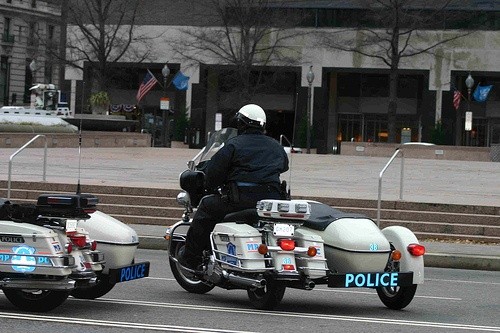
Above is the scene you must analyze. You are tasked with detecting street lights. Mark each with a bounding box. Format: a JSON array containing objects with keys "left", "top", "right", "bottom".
[
  {"left": 463, "top": 74, "right": 475, "bottom": 151},
  {"left": 304, "top": 70, "right": 315, "bottom": 154},
  {"left": 159, "top": 64, "right": 171, "bottom": 147},
  {"left": 30, "top": 60, "right": 37, "bottom": 94}
]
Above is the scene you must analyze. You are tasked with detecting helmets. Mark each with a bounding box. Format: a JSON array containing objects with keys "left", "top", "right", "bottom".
[{"left": 232, "top": 104, "right": 266, "bottom": 130}]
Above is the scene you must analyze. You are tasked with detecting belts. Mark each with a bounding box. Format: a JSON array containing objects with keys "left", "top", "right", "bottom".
[{"left": 236, "top": 185, "right": 277, "bottom": 190}]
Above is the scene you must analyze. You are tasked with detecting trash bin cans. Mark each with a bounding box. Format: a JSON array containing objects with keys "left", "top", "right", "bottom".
[{"left": 184, "top": 125, "right": 203, "bottom": 149}]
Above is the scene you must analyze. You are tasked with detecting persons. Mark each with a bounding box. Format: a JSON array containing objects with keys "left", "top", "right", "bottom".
[{"left": 177, "top": 103, "right": 290, "bottom": 274}]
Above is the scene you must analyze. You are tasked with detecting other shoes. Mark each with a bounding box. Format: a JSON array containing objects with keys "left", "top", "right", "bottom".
[{"left": 178, "top": 246, "right": 192, "bottom": 264}]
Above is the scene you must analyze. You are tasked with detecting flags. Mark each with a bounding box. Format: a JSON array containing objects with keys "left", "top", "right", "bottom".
[
  {"left": 135, "top": 72, "right": 157, "bottom": 102},
  {"left": 172, "top": 71, "right": 190, "bottom": 91},
  {"left": 473, "top": 85, "right": 495, "bottom": 103},
  {"left": 453, "top": 88, "right": 462, "bottom": 110}
]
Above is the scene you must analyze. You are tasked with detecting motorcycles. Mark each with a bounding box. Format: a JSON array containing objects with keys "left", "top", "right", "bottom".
[
  {"left": 164, "top": 127, "right": 426, "bottom": 311},
  {"left": 0, "top": 76, "right": 150, "bottom": 313}
]
